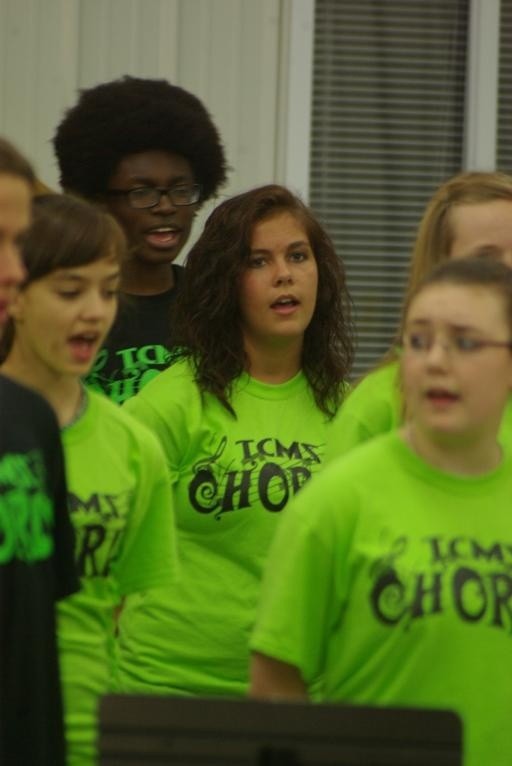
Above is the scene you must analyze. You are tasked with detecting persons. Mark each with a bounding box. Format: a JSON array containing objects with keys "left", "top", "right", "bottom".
[
  {"left": 246, "top": 255, "right": 510, "bottom": 765},
  {"left": 323, "top": 170, "right": 511, "bottom": 465},
  {"left": 109, "top": 183, "right": 355, "bottom": 699},
  {"left": 1, "top": 192, "right": 181, "bottom": 766},
  {"left": 52, "top": 75, "right": 228, "bottom": 407},
  {"left": 0, "top": 133, "right": 84, "bottom": 766}
]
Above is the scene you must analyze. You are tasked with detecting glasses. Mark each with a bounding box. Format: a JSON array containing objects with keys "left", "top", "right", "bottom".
[
  {"left": 394, "top": 336, "right": 512, "bottom": 359},
  {"left": 111, "top": 183, "right": 202, "bottom": 210}
]
